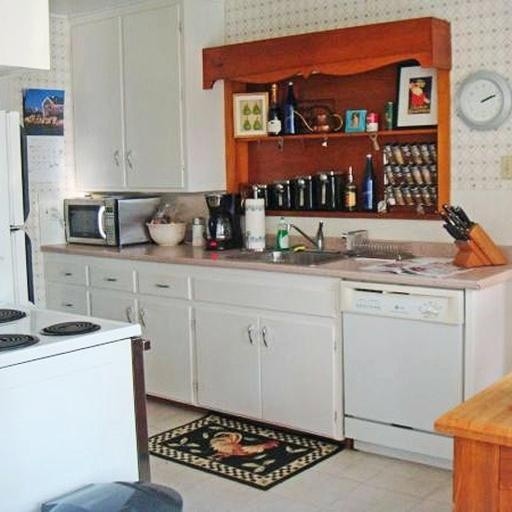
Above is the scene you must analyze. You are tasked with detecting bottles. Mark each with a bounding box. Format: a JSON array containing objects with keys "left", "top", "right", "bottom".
[
  {"left": 267, "top": 84, "right": 281, "bottom": 137},
  {"left": 283, "top": 83, "right": 297, "bottom": 134},
  {"left": 191, "top": 217, "right": 206, "bottom": 247},
  {"left": 343, "top": 165, "right": 358, "bottom": 213},
  {"left": 360, "top": 153, "right": 378, "bottom": 210},
  {"left": 277, "top": 218, "right": 291, "bottom": 252},
  {"left": 382, "top": 140, "right": 438, "bottom": 208}
]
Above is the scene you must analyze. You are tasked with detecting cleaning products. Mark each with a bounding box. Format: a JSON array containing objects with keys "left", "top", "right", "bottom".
[{"left": 276, "top": 214, "right": 291, "bottom": 250}]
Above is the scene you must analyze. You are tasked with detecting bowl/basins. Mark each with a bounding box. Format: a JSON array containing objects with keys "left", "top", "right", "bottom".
[{"left": 145, "top": 222, "right": 187, "bottom": 247}]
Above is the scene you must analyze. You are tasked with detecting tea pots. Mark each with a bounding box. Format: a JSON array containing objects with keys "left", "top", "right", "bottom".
[{"left": 294, "top": 103, "right": 344, "bottom": 133}]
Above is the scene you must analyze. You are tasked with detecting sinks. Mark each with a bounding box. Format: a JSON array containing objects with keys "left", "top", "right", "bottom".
[{"left": 226, "top": 248, "right": 350, "bottom": 270}]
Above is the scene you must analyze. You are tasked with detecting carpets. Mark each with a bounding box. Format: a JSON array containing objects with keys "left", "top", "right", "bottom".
[{"left": 148, "top": 413, "right": 346, "bottom": 490}]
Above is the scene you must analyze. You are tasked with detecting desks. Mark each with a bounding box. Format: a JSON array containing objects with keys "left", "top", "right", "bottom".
[{"left": 435, "top": 372, "right": 512, "bottom": 512}]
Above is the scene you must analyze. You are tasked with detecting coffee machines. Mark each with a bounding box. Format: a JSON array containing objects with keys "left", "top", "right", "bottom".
[{"left": 206, "top": 192, "right": 244, "bottom": 252}]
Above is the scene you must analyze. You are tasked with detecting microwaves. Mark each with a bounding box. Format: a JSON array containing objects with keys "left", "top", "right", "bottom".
[{"left": 63, "top": 194, "right": 161, "bottom": 249}]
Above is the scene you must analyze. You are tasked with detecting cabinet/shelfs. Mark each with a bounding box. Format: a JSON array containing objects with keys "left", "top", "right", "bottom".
[
  {"left": 71, "top": 0, "right": 228, "bottom": 193},
  {"left": 204, "top": 17, "right": 452, "bottom": 221}
]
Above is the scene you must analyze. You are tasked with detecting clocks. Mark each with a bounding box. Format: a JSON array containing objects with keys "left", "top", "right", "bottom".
[{"left": 458, "top": 71, "right": 511, "bottom": 131}]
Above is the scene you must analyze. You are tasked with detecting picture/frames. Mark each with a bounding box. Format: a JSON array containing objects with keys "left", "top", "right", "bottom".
[
  {"left": 232, "top": 91, "right": 269, "bottom": 138},
  {"left": 393, "top": 64, "right": 437, "bottom": 130}
]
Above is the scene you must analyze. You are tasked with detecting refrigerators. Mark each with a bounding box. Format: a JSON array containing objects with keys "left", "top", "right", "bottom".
[{"left": 0, "top": 109, "right": 35, "bottom": 309}]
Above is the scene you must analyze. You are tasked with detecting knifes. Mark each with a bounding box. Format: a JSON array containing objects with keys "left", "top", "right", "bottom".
[{"left": 439, "top": 204, "right": 476, "bottom": 241}]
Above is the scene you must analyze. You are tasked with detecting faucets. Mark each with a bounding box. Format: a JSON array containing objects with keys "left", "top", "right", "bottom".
[{"left": 289, "top": 222, "right": 327, "bottom": 252}]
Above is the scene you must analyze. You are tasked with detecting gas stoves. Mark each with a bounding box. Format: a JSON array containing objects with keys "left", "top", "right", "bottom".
[{"left": 0, "top": 304, "right": 134, "bottom": 357}]
